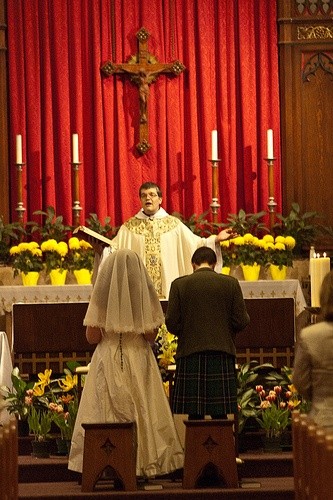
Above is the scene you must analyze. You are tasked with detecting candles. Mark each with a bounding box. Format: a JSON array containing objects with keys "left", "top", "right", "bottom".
[
  {"left": 268, "top": 129, "right": 274, "bottom": 159},
  {"left": 73, "top": 134, "right": 79, "bottom": 163},
  {"left": 16, "top": 135, "right": 22, "bottom": 163},
  {"left": 212, "top": 130, "right": 218, "bottom": 160},
  {"left": 309, "top": 246, "right": 331, "bottom": 307}
]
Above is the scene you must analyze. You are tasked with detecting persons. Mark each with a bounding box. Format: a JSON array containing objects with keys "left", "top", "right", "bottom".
[
  {"left": 165, "top": 247, "right": 252, "bottom": 419},
  {"left": 68, "top": 248, "right": 187, "bottom": 476},
  {"left": 117, "top": 63, "right": 172, "bottom": 123},
  {"left": 292, "top": 270, "right": 333, "bottom": 428},
  {"left": 84, "top": 182, "right": 239, "bottom": 300}
]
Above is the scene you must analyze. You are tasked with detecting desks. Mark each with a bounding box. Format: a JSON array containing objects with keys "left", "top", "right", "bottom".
[{"left": 0, "top": 280, "right": 307, "bottom": 348}]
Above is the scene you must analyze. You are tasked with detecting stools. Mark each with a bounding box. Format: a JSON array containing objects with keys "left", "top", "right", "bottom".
[
  {"left": 82, "top": 422, "right": 138, "bottom": 492},
  {"left": 183, "top": 419, "right": 238, "bottom": 490}
]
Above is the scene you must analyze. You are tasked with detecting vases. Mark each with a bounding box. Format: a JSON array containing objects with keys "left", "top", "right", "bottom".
[
  {"left": 73, "top": 268, "right": 94, "bottom": 284},
  {"left": 49, "top": 269, "right": 67, "bottom": 285},
  {"left": 21, "top": 271, "right": 39, "bottom": 286},
  {"left": 31, "top": 436, "right": 70, "bottom": 458},
  {"left": 221, "top": 264, "right": 287, "bottom": 282},
  {"left": 243, "top": 431, "right": 281, "bottom": 454}
]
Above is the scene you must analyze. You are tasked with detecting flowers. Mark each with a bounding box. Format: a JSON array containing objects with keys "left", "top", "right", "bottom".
[
  {"left": 9, "top": 237, "right": 96, "bottom": 278},
  {"left": 219, "top": 234, "right": 296, "bottom": 271},
  {"left": 0, "top": 360, "right": 306, "bottom": 439}
]
